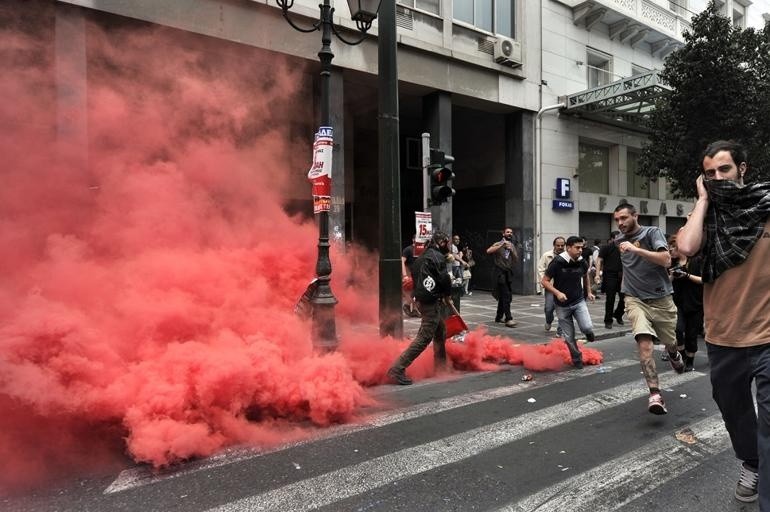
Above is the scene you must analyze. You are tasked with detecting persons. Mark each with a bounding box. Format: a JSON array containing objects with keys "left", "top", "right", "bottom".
[
  {"left": 401, "top": 234, "right": 423, "bottom": 317},
  {"left": 536, "top": 237, "right": 566, "bottom": 338},
  {"left": 676, "top": 140, "right": 769, "bottom": 512},
  {"left": 662, "top": 233, "right": 705, "bottom": 371},
  {"left": 594, "top": 231, "right": 626, "bottom": 329},
  {"left": 580, "top": 236, "right": 613, "bottom": 299},
  {"left": 388, "top": 232, "right": 453, "bottom": 386},
  {"left": 486, "top": 227, "right": 518, "bottom": 327},
  {"left": 451, "top": 235, "right": 475, "bottom": 295},
  {"left": 541, "top": 236, "right": 597, "bottom": 370},
  {"left": 613, "top": 203, "right": 685, "bottom": 415}
]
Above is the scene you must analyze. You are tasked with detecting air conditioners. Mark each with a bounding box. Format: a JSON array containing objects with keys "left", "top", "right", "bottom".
[{"left": 493, "top": 38, "right": 522, "bottom": 66}]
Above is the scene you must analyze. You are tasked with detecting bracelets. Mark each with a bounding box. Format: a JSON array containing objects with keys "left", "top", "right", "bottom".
[{"left": 684, "top": 273, "right": 690, "bottom": 278}]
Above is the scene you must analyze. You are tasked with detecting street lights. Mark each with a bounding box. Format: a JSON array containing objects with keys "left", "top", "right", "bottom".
[{"left": 273, "top": 1, "right": 385, "bottom": 359}]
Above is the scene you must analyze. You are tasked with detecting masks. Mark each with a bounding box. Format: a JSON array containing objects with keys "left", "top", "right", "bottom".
[{"left": 438, "top": 241, "right": 449, "bottom": 255}]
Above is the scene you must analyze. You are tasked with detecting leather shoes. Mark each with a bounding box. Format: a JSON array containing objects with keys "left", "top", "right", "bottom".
[
  {"left": 388, "top": 368, "right": 412, "bottom": 384},
  {"left": 495, "top": 317, "right": 516, "bottom": 325},
  {"left": 605, "top": 313, "right": 624, "bottom": 329}
]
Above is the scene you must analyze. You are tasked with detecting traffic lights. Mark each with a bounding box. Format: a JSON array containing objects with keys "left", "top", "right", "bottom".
[
  {"left": 430, "top": 148, "right": 456, "bottom": 198},
  {"left": 429, "top": 165, "right": 451, "bottom": 206}
]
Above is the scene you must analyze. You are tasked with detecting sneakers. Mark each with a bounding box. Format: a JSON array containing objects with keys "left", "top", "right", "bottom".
[
  {"left": 648, "top": 393, "right": 667, "bottom": 415},
  {"left": 734, "top": 462, "right": 759, "bottom": 502},
  {"left": 661, "top": 351, "right": 695, "bottom": 373}
]
